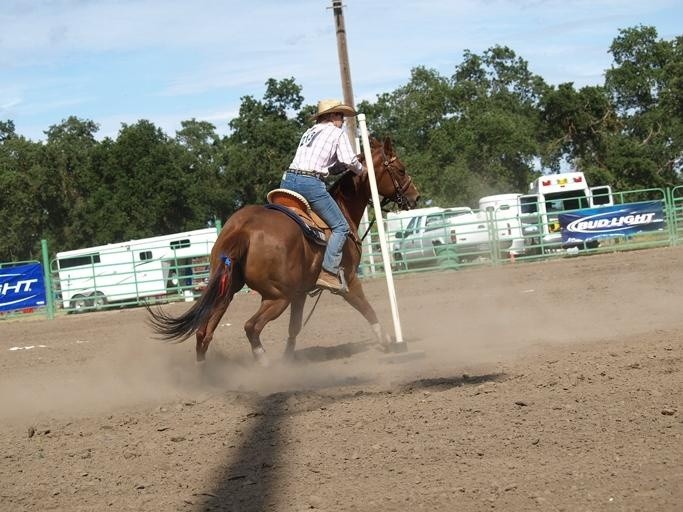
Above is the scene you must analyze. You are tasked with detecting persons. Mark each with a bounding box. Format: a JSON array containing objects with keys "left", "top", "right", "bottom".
[{"left": 278, "top": 99, "right": 370, "bottom": 295}]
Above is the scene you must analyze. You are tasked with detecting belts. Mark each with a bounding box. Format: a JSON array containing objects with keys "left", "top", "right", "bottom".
[{"left": 286, "top": 168, "right": 324, "bottom": 182}]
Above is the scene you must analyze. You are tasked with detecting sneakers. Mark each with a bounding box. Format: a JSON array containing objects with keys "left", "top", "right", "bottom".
[{"left": 316, "top": 268, "right": 350, "bottom": 293}]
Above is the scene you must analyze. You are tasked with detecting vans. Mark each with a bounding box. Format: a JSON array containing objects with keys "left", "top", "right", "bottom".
[{"left": 516, "top": 171, "right": 614, "bottom": 255}]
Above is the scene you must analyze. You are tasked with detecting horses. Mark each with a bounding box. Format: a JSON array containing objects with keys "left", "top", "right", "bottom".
[{"left": 142, "top": 132, "right": 421, "bottom": 392}]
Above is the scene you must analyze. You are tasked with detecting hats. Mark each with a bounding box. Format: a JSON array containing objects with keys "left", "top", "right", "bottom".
[{"left": 307, "top": 99, "right": 356, "bottom": 122}]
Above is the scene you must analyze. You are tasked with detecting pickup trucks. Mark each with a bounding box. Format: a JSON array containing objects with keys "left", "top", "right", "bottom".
[{"left": 393, "top": 206, "right": 514, "bottom": 268}]
